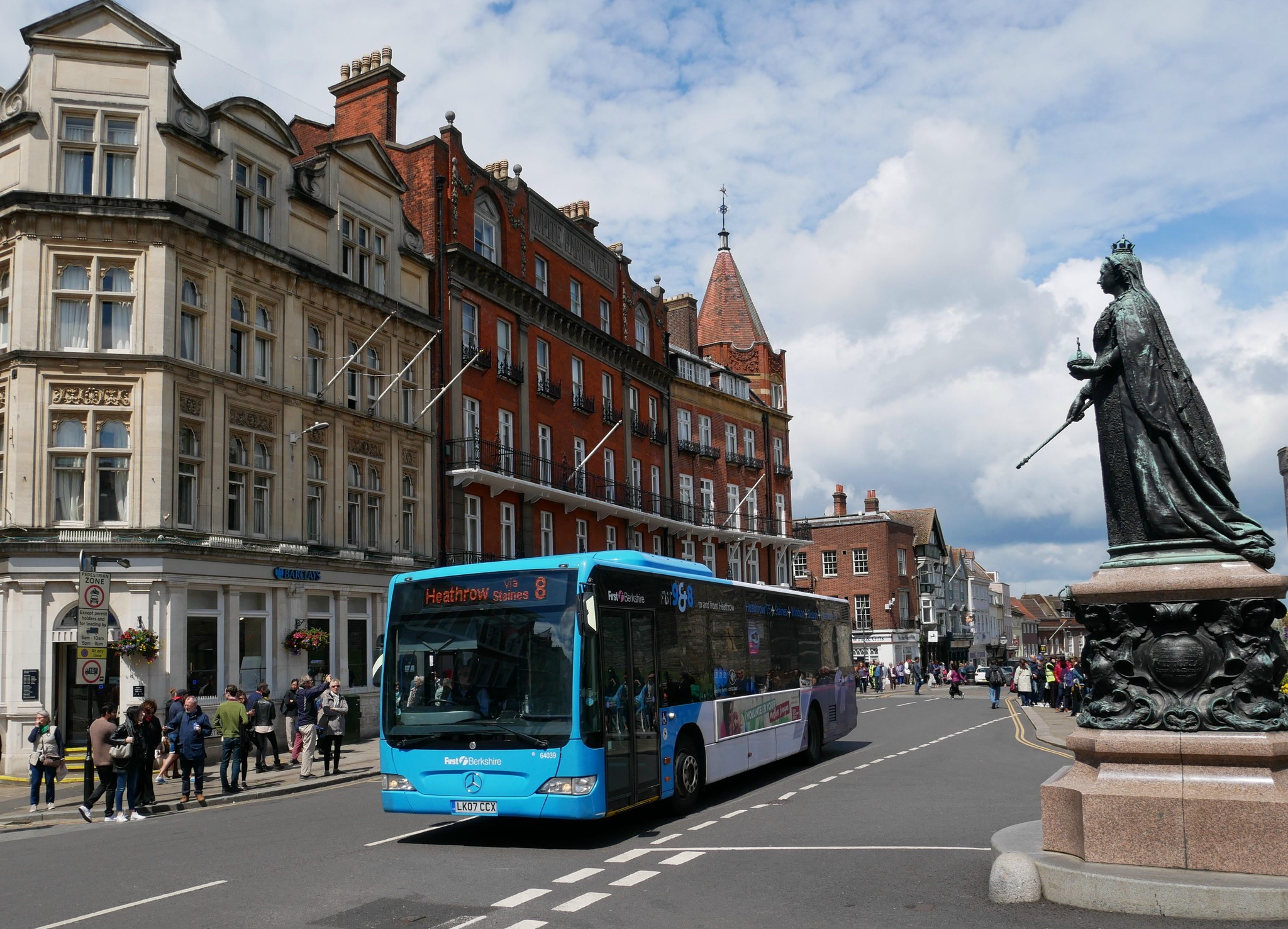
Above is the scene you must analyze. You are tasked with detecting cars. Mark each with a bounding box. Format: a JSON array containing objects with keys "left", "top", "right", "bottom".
[
  {"left": 1001, "top": 666, "right": 1015, "bottom": 681},
  {"left": 975, "top": 666, "right": 1011, "bottom": 686}
]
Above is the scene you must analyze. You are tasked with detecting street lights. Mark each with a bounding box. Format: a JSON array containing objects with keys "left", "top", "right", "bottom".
[
  {"left": 83, "top": 555, "right": 131, "bottom": 806},
  {"left": 859, "top": 604, "right": 866, "bottom": 669},
  {"left": 1002, "top": 606, "right": 1019, "bottom": 636}
]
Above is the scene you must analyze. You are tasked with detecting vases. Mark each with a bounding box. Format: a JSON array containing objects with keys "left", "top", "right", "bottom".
[{"left": 301, "top": 645, "right": 315, "bottom": 651}]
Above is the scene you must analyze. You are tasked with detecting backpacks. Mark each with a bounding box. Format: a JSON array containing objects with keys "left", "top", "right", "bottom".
[{"left": 990, "top": 666, "right": 1002, "bottom": 687}]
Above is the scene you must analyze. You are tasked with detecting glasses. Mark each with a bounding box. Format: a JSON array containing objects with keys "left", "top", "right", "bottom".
[
  {"left": 224, "top": 692, "right": 228, "bottom": 695},
  {"left": 146, "top": 711, "right": 155, "bottom": 715},
  {"left": 395, "top": 684, "right": 399, "bottom": 687},
  {"left": 736, "top": 673, "right": 742, "bottom": 675},
  {"left": 239, "top": 697, "right": 246, "bottom": 700},
  {"left": 35, "top": 717, "right": 47, "bottom": 720},
  {"left": 306, "top": 679, "right": 312, "bottom": 683},
  {"left": 332, "top": 685, "right": 340, "bottom": 688},
  {"left": 648, "top": 676, "right": 654, "bottom": 679},
  {"left": 458, "top": 672, "right": 476, "bottom": 677}
]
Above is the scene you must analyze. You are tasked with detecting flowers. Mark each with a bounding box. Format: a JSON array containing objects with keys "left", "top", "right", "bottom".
[
  {"left": 281, "top": 627, "right": 331, "bottom": 656},
  {"left": 110, "top": 628, "right": 166, "bottom": 667}
]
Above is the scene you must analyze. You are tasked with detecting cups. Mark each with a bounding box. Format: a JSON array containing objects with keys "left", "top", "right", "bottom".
[{"left": 193, "top": 723, "right": 199, "bottom": 730}]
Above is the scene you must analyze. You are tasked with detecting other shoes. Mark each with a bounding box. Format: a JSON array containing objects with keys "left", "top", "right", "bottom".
[
  {"left": 1017, "top": 693, "right": 1082, "bottom": 717},
  {"left": 858, "top": 681, "right": 965, "bottom": 700},
  {"left": 135, "top": 749, "right": 345, "bottom": 807}
]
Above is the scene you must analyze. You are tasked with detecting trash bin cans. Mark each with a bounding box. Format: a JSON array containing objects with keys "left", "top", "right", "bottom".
[{"left": 965, "top": 669, "right": 977, "bottom": 685}]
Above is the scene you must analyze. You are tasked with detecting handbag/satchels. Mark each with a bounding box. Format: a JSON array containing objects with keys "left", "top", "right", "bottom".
[
  {"left": 315, "top": 725, "right": 324, "bottom": 738},
  {"left": 174, "top": 735, "right": 180, "bottom": 754},
  {"left": 246, "top": 702, "right": 257, "bottom": 737},
  {"left": 43, "top": 753, "right": 61, "bottom": 768},
  {"left": 280, "top": 689, "right": 291, "bottom": 715},
  {"left": 154, "top": 737, "right": 170, "bottom": 759},
  {"left": 1009, "top": 681, "right": 1018, "bottom": 692},
  {"left": 891, "top": 674, "right": 896, "bottom": 677},
  {"left": 961, "top": 669, "right": 966, "bottom": 673},
  {"left": 899, "top": 672, "right": 904, "bottom": 676},
  {"left": 109, "top": 723, "right": 132, "bottom": 759},
  {"left": 56, "top": 764, "right": 69, "bottom": 782},
  {"left": 942, "top": 666, "right": 947, "bottom": 675}
]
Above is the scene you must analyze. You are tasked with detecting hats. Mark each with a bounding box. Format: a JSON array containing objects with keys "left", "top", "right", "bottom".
[{"left": 173, "top": 689, "right": 187, "bottom": 700}]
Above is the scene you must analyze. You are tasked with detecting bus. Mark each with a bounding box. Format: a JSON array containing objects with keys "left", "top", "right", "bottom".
[
  {"left": 1004, "top": 657, "right": 1029, "bottom": 672},
  {"left": 375, "top": 550, "right": 857, "bottom": 823}
]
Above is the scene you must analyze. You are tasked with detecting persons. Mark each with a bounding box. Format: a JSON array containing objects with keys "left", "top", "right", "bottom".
[
  {"left": 28, "top": 710, "right": 65, "bottom": 812},
  {"left": 603, "top": 667, "right": 815, "bottom": 734},
  {"left": 162, "top": 696, "right": 212, "bottom": 803},
  {"left": 78, "top": 702, "right": 118, "bottom": 823},
  {"left": 1067, "top": 234, "right": 1276, "bottom": 552},
  {"left": 395, "top": 675, "right": 452, "bottom": 716},
  {"left": 855, "top": 656, "right": 981, "bottom": 699},
  {"left": 155, "top": 688, "right": 195, "bottom": 784},
  {"left": 107, "top": 706, "right": 147, "bottom": 823},
  {"left": 442, "top": 665, "right": 492, "bottom": 720},
  {"left": 134, "top": 698, "right": 162, "bottom": 807},
  {"left": 1014, "top": 654, "right": 1091, "bottom": 716},
  {"left": 213, "top": 674, "right": 349, "bottom": 794},
  {"left": 985, "top": 660, "right": 1007, "bottom": 709}
]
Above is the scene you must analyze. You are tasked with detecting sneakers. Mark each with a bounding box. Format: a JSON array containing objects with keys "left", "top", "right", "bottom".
[{"left": 29, "top": 801, "right": 146, "bottom": 823}]
